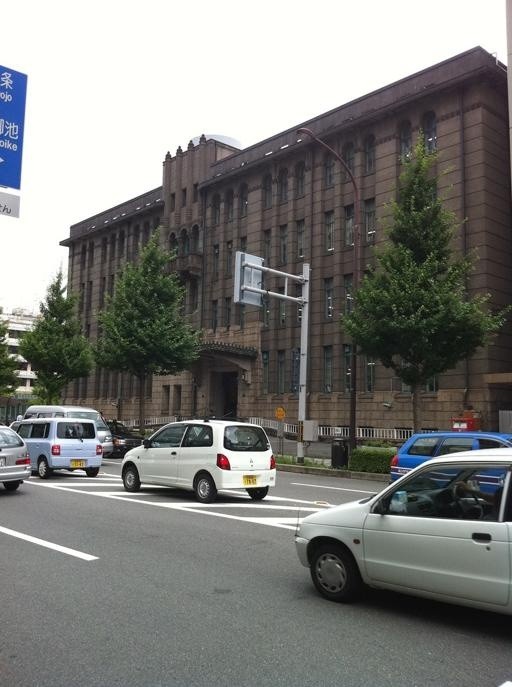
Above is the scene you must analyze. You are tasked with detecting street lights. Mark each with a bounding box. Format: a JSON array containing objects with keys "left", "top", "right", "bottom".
[{"left": 297, "top": 127, "right": 359, "bottom": 447}]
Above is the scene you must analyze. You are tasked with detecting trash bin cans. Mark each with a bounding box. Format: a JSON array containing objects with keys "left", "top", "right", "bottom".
[{"left": 331, "top": 438, "right": 348, "bottom": 468}]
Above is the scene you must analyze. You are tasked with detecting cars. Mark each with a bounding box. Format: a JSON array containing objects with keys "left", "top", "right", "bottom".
[
  {"left": 294, "top": 446, "right": 512, "bottom": 614},
  {"left": 0, "top": 424, "right": 31, "bottom": 493},
  {"left": 121, "top": 416, "right": 276, "bottom": 502},
  {"left": 106, "top": 420, "right": 144, "bottom": 458}
]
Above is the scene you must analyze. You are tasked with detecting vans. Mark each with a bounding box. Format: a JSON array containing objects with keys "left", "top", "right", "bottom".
[
  {"left": 17, "top": 405, "right": 114, "bottom": 459},
  {"left": 8, "top": 418, "right": 104, "bottom": 478},
  {"left": 390, "top": 432, "right": 512, "bottom": 492}
]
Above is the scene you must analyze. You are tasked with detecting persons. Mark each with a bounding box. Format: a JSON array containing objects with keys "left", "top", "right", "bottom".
[
  {"left": 454, "top": 478, "right": 498, "bottom": 504},
  {"left": 16, "top": 412, "right": 22, "bottom": 421}
]
